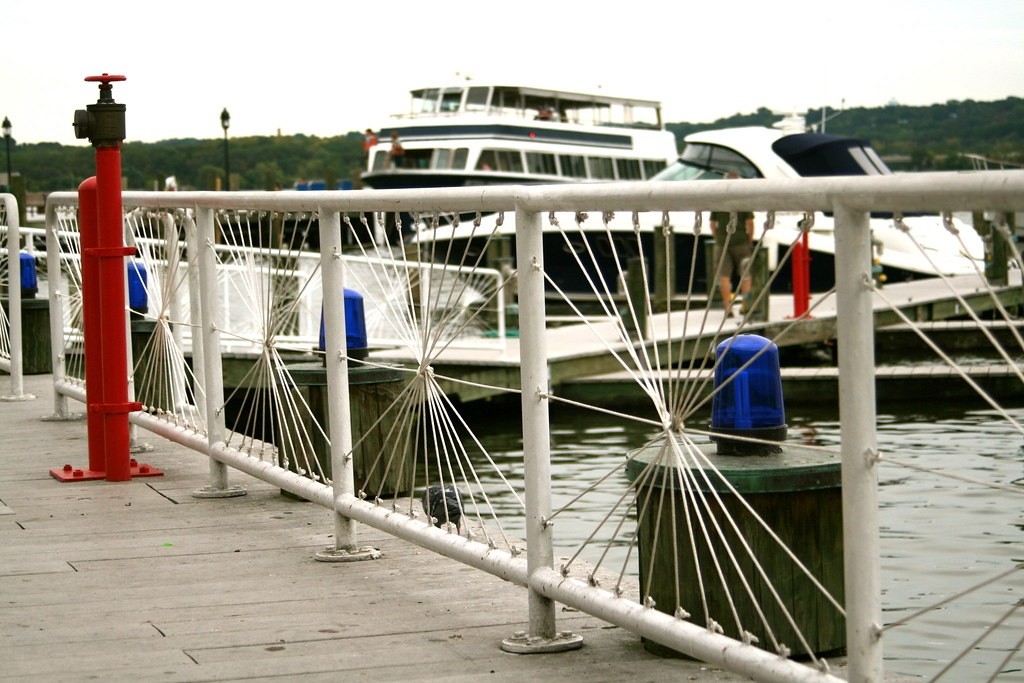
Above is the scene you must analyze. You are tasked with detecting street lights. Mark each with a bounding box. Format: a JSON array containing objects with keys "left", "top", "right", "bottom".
[
  {"left": 221, "top": 109, "right": 232, "bottom": 192},
  {"left": 2, "top": 116, "right": 13, "bottom": 194}
]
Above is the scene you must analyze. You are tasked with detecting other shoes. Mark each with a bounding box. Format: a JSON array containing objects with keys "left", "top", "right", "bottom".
[
  {"left": 727, "top": 312, "right": 733, "bottom": 317},
  {"left": 739, "top": 308, "right": 746, "bottom": 314}
]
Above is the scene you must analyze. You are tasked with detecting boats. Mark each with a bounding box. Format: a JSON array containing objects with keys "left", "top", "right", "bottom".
[{"left": 362, "top": 77, "right": 1024, "bottom": 420}]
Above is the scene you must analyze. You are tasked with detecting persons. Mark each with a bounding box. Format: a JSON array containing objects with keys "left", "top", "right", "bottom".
[
  {"left": 709, "top": 171, "right": 755, "bottom": 319},
  {"left": 384, "top": 131, "right": 404, "bottom": 168},
  {"left": 360, "top": 129, "right": 377, "bottom": 170},
  {"left": 533, "top": 107, "right": 557, "bottom": 121}
]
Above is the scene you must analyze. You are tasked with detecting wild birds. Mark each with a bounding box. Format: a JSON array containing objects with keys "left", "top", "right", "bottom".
[{"left": 421, "top": 484, "right": 464, "bottom": 541}]
